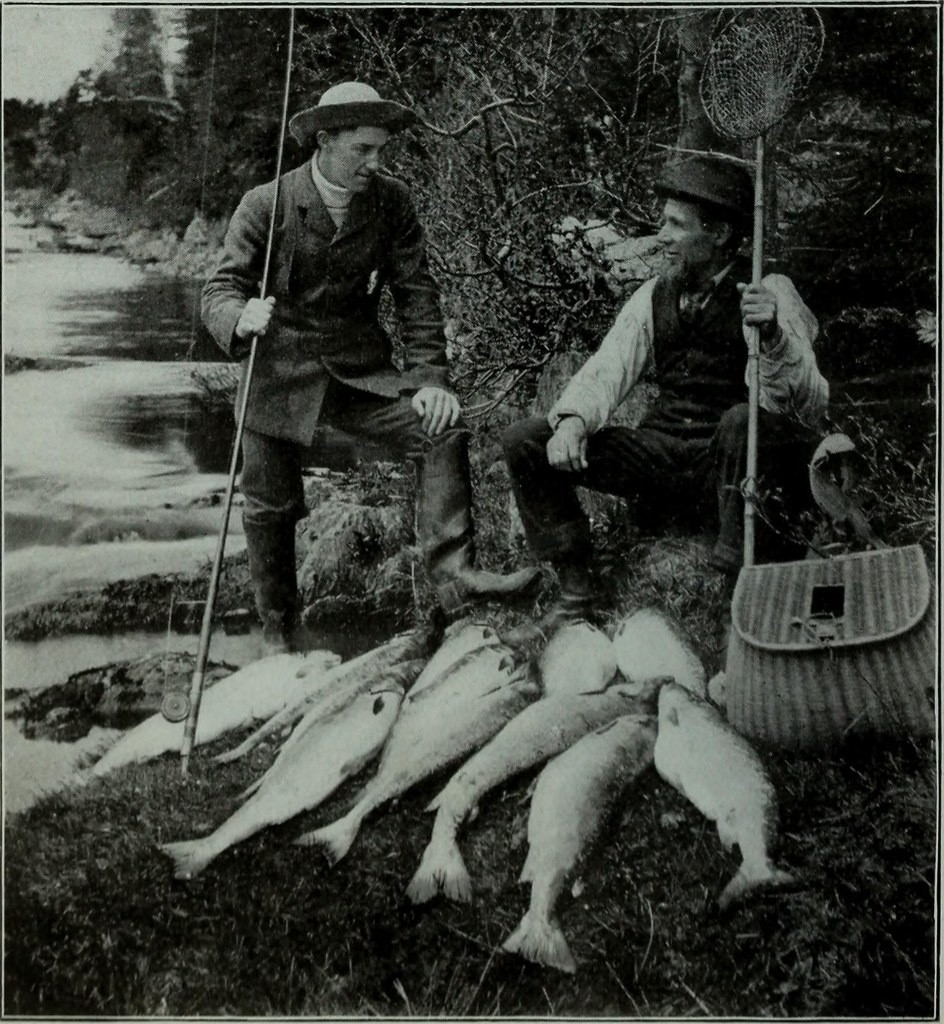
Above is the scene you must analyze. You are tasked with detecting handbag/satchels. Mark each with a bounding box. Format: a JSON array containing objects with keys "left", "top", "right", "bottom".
[{"left": 724, "top": 433, "right": 936, "bottom": 753}]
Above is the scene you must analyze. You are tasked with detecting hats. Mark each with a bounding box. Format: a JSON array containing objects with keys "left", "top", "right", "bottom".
[
  {"left": 289, "top": 81, "right": 417, "bottom": 157},
  {"left": 651, "top": 153, "right": 768, "bottom": 239}
]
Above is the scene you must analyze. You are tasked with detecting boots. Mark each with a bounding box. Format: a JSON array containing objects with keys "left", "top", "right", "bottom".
[
  {"left": 242, "top": 510, "right": 303, "bottom": 658},
  {"left": 407, "top": 428, "right": 543, "bottom": 625},
  {"left": 500, "top": 515, "right": 616, "bottom": 649}
]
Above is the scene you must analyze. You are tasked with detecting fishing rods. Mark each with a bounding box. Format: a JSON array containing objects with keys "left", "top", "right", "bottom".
[{"left": 177, "top": 9, "right": 294, "bottom": 778}]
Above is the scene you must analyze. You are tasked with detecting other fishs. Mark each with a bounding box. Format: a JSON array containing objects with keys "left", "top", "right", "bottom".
[
  {"left": 78, "top": 604, "right": 705, "bottom": 977},
  {"left": 653, "top": 680, "right": 796, "bottom": 906}
]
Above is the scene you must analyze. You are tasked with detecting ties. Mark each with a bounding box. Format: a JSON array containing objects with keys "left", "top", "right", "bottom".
[{"left": 680, "top": 294, "right": 710, "bottom": 324}]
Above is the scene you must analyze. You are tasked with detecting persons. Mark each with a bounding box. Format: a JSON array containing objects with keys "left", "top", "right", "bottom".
[
  {"left": 198, "top": 80, "right": 543, "bottom": 646},
  {"left": 503, "top": 154, "right": 829, "bottom": 649}
]
